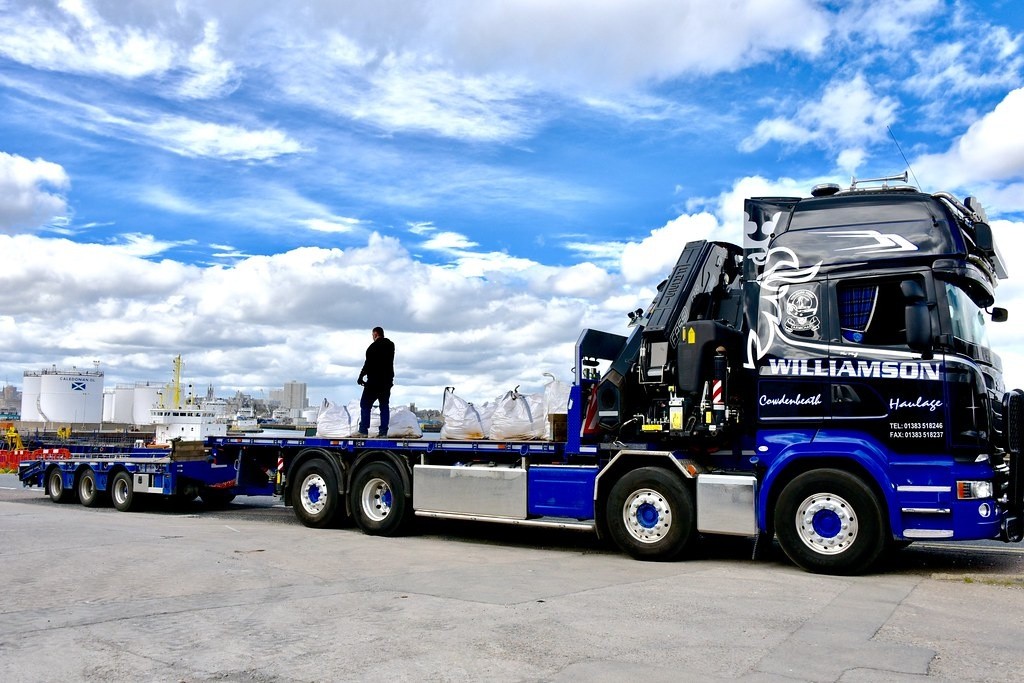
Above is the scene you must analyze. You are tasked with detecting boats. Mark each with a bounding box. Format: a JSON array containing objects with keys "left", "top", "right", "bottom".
[{"left": 227, "top": 416, "right": 264, "bottom": 433}]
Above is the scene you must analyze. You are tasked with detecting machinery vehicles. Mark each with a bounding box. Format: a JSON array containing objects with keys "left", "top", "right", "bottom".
[{"left": 260, "top": 388, "right": 270, "bottom": 418}]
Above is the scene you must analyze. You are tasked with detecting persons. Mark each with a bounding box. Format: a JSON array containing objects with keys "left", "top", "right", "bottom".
[{"left": 350, "top": 327, "right": 395, "bottom": 438}]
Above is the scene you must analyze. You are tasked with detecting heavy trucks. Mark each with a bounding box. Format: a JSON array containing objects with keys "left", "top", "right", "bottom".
[{"left": 20, "top": 172, "right": 1024, "bottom": 577}]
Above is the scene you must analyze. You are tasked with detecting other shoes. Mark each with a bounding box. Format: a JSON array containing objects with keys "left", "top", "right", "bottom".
[
  {"left": 374, "top": 434, "right": 387, "bottom": 440},
  {"left": 351, "top": 431, "right": 368, "bottom": 438}
]
